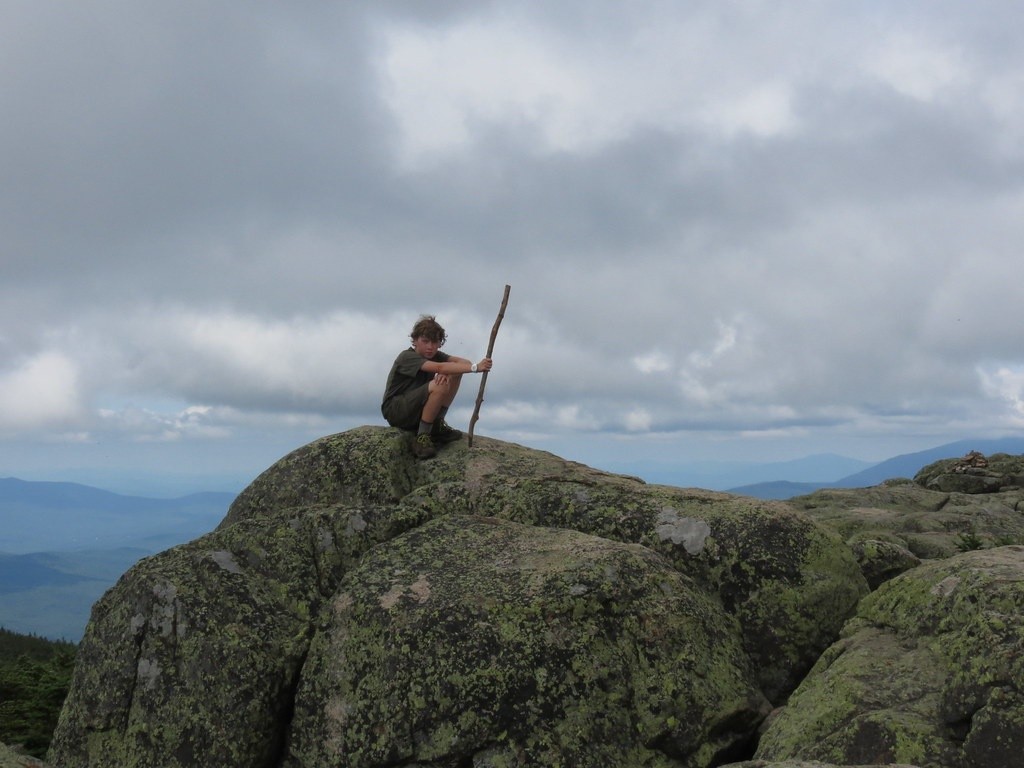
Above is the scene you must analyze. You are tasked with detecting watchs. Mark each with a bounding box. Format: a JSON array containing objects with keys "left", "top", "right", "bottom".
[{"left": 471, "top": 363, "right": 478, "bottom": 373}]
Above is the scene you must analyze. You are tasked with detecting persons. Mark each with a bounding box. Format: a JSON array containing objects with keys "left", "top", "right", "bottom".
[{"left": 381, "top": 314, "right": 492, "bottom": 459}]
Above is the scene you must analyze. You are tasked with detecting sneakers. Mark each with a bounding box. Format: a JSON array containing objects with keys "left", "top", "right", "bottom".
[{"left": 410, "top": 422, "right": 463, "bottom": 458}]
